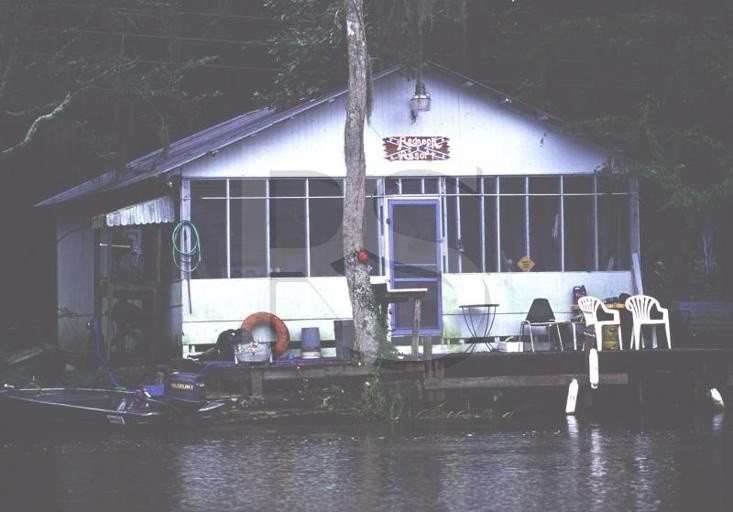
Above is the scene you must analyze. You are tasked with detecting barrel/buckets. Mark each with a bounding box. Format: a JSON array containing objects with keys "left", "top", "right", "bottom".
[{"left": 300, "top": 327, "right": 323, "bottom": 358}]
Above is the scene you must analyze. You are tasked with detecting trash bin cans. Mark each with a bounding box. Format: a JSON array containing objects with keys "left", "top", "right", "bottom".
[{"left": 334, "top": 320, "right": 355, "bottom": 361}]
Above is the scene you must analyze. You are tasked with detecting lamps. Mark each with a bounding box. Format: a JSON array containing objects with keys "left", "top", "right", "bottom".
[{"left": 410, "top": 78, "right": 432, "bottom": 121}]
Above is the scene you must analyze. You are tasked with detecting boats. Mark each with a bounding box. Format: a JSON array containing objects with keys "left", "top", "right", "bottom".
[{"left": 0, "top": 386, "right": 225, "bottom": 426}]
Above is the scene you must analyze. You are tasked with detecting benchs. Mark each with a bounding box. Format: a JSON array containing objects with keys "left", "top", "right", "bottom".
[{"left": 672, "top": 298, "right": 732, "bottom": 348}]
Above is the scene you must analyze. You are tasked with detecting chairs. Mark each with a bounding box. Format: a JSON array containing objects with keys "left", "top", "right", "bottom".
[{"left": 518, "top": 293, "right": 672, "bottom": 352}]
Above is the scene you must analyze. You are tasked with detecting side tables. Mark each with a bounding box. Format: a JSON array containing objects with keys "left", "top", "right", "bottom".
[{"left": 459, "top": 303, "right": 500, "bottom": 352}]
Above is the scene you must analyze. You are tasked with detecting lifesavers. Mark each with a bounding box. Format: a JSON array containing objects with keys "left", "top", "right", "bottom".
[{"left": 240, "top": 312, "right": 290, "bottom": 358}]
